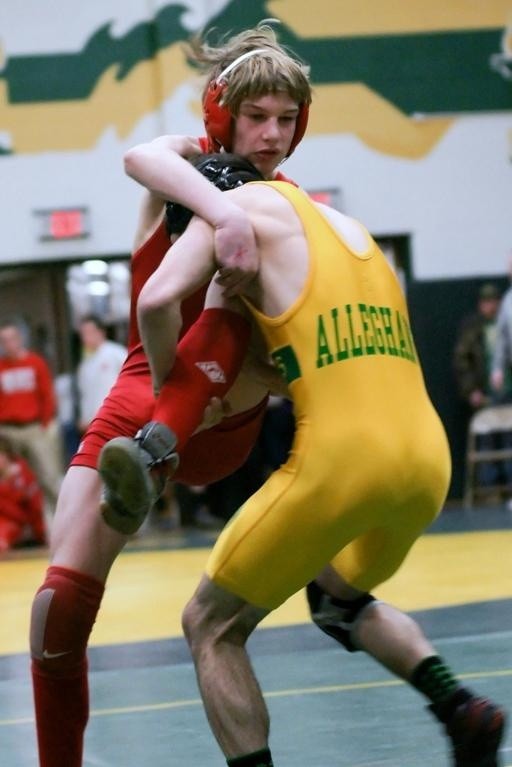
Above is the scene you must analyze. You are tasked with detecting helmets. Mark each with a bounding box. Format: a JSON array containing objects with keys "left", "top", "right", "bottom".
[{"left": 165, "top": 152, "right": 266, "bottom": 234}]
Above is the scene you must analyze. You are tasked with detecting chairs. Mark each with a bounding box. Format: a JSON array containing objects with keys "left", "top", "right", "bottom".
[{"left": 462, "top": 403, "right": 511, "bottom": 514}]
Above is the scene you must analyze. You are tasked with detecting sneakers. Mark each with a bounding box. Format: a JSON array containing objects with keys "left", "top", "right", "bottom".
[
  {"left": 96, "top": 419, "right": 181, "bottom": 535},
  {"left": 423, "top": 685, "right": 505, "bottom": 766}
]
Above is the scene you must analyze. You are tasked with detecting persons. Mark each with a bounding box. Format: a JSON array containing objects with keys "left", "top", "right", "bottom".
[
  {"left": 0, "top": 439, "right": 48, "bottom": 558},
  {"left": 74, "top": 313, "right": 128, "bottom": 430},
  {"left": 1, "top": 318, "right": 66, "bottom": 506},
  {"left": 136, "top": 150, "right": 512, "bottom": 766},
  {"left": 490, "top": 286, "right": 510, "bottom": 387},
  {"left": 454, "top": 282, "right": 511, "bottom": 508},
  {"left": 30, "top": 15, "right": 317, "bottom": 766}
]
